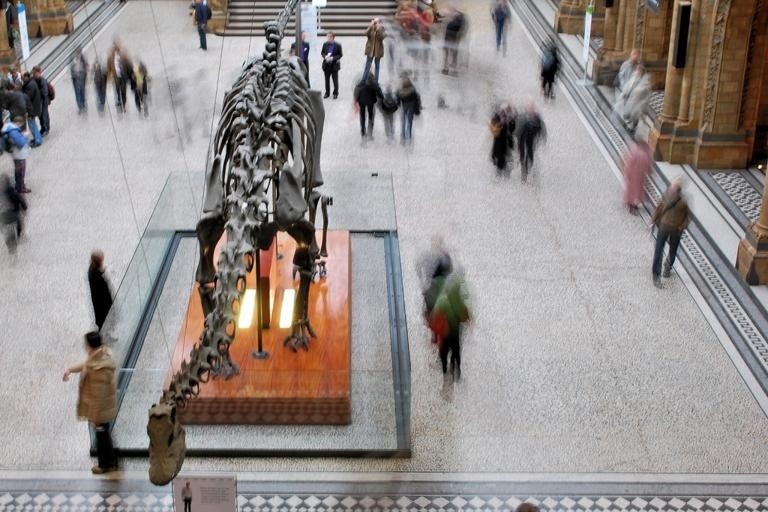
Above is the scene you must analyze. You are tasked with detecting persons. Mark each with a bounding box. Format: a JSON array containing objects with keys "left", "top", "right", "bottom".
[
  {"left": 615, "top": 64, "right": 654, "bottom": 134},
  {"left": 85, "top": 249, "right": 114, "bottom": 328},
  {"left": 647, "top": 177, "right": 691, "bottom": 286},
  {"left": 428, "top": 271, "right": 469, "bottom": 385},
  {"left": 539, "top": 36, "right": 563, "bottom": 100},
  {"left": 289, "top": 30, "right": 312, "bottom": 89},
  {"left": 350, "top": 1, "right": 466, "bottom": 148},
  {"left": 414, "top": 235, "right": 454, "bottom": 342},
  {"left": 515, "top": 101, "right": 550, "bottom": 184},
  {"left": 180, "top": 480, "right": 193, "bottom": 512},
  {"left": 614, "top": 49, "right": 641, "bottom": 119},
  {"left": 491, "top": 0, "right": 514, "bottom": 55},
  {"left": 60, "top": 331, "right": 120, "bottom": 473},
  {"left": 623, "top": 134, "right": 653, "bottom": 216},
  {"left": 0, "top": 37, "right": 150, "bottom": 259},
  {"left": 320, "top": 31, "right": 343, "bottom": 98},
  {"left": 489, "top": 103, "right": 517, "bottom": 180},
  {"left": 189, "top": 0, "right": 211, "bottom": 48}
]
